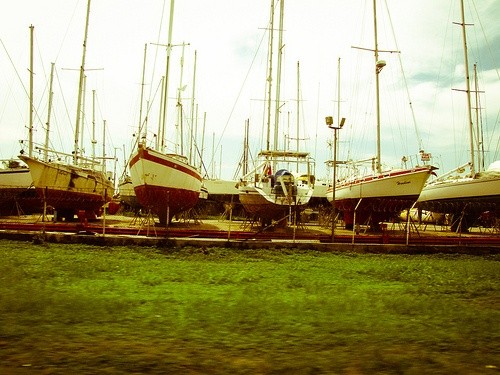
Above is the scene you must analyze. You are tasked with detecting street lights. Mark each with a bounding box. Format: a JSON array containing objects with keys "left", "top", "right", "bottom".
[{"left": 325, "top": 116, "right": 346, "bottom": 242}]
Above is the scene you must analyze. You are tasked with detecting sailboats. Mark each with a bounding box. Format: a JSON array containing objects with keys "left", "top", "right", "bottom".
[
  {"left": 325, "top": 0, "right": 440, "bottom": 227},
  {"left": 410, "top": 0, "right": 500, "bottom": 226},
  {"left": 15, "top": 0, "right": 117, "bottom": 215},
  {"left": 233, "top": 0, "right": 317, "bottom": 223},
  {"left": 0, "top": 60, "right": 330, "bottom": 217},
  {"left": 129, "top": 0, "right": 206, "bottom": 225}
]
